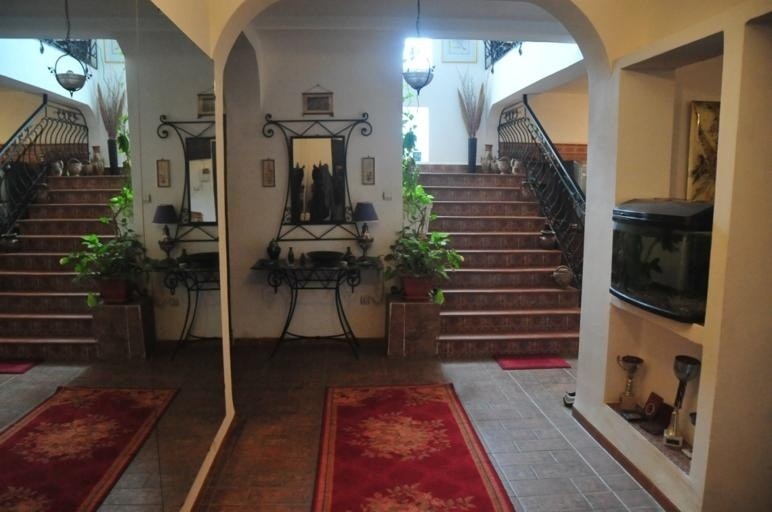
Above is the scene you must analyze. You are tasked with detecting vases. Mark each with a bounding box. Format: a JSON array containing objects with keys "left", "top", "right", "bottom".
[
  {"left": 468, "top": 139, "right": 476, "bottom": 173},
  {"left": 42, "top": 139, "right": 106, "bottom": 179},
  {"left": 481, "top": 143, "right": 527, "bottom": 176}
]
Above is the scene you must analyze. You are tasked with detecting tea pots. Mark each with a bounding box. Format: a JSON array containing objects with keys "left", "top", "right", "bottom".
[
  {"left": 49, "top": 159, "right": 64, "bottom": 177},
  {"left": 510, "top": 158, "right": 526, "bottom": 175}
]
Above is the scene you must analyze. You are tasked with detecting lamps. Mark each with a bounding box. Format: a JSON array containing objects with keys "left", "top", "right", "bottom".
[
  {"left": 151, "top": 205, "right": 181, "bottom": 260},
  {"left": 51, "top": 4, "right": 93, "bottom": 98},
  {"left": 192, "top": 159, "right": 210, "bottom": 192},
  {"left": 401, "top": 1, "right": 437, "bottom": 98},
  {"left": 353, "top": 202, "right": 378, "bottom": 264}
]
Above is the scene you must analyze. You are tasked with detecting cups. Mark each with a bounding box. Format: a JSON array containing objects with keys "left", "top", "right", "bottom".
[{"left": 67, "top": 163, "right": 82, "bottom": 176}]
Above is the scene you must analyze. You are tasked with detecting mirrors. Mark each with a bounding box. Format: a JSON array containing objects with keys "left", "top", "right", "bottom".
[
  {"left": 263, "top": 113, "right": 372, "bottom": 261},
  {"left": 156, "top": 113, "right": 222, "bottom": 244},
  {"left": 0, "top": 1, "right": 235, "bottom": 512}
]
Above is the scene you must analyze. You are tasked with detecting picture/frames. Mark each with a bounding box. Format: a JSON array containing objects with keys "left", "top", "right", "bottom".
[
  {"left": 441, "top": 39, "right": 478, "bottom": 64},
  {"left": 104, "top": 38, "right": 123, "bottom": 64}
]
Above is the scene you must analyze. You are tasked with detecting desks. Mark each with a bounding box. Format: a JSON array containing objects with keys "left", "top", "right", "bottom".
[
  {"left": 252, "top": 254, "right": 382, "bottom": 361},
  {"left": 160, "top": 249, "right": 224, "bottom": 356}
]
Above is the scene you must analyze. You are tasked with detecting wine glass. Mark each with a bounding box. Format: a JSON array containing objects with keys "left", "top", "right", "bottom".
[{"left": 496, "top": 159, "right": 509, "bottom": 175}]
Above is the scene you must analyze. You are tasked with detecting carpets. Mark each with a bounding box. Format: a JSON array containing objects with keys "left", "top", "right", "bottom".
[
  {"left": 0, "top": 385, "right": 181, "bottom": 512},
  {"left": 2, "top": 357, "right": 36, "bottom": 373},
  {"left": 312, "top": 382, "right": 517, "bottom": 511},
  {"left": 495, "top": 354, "right": 573, "bottom": 369}
]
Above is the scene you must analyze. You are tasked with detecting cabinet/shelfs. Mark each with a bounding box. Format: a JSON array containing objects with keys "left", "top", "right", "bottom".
[{"left": 601, "top": 23, "right": 745, "bottom": 506}]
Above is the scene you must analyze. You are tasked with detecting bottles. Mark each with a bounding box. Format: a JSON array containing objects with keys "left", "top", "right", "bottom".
[
  {"left": 90, "top": 145, "right": 105, "bottom": 174},
  {"left": 287, "top": 246, "right": 295, "bottom": 264},
  {"left": 266, "top": 238, "right": 281, "bottom": 261},
  {"left": 298, "top": 251, "right": 307, "bottom": 266},
  {"left": 479, "top": 144, "right": 492, "bottom": 172},
  {"left": 341, "top": 246, "right": 357, "bottom": 265},
  {"left": 177, "top": 247, "right": 189, "bottom": 264}
]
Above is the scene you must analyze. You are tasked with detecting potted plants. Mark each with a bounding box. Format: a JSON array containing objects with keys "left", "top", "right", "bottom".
[
  {"left": 60, "top": 68, "right": 155, "bottom": 309},
  {"left": 377, "top": 82, "right": 468, "bottom": 300}
]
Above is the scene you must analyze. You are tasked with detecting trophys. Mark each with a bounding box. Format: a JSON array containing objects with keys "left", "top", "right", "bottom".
[
  {"left": 615, "top": 353, "right": 646, "bottom": 411},
  {"left": 663, "top": 354, "right": 701, "bottom": 449}
]
